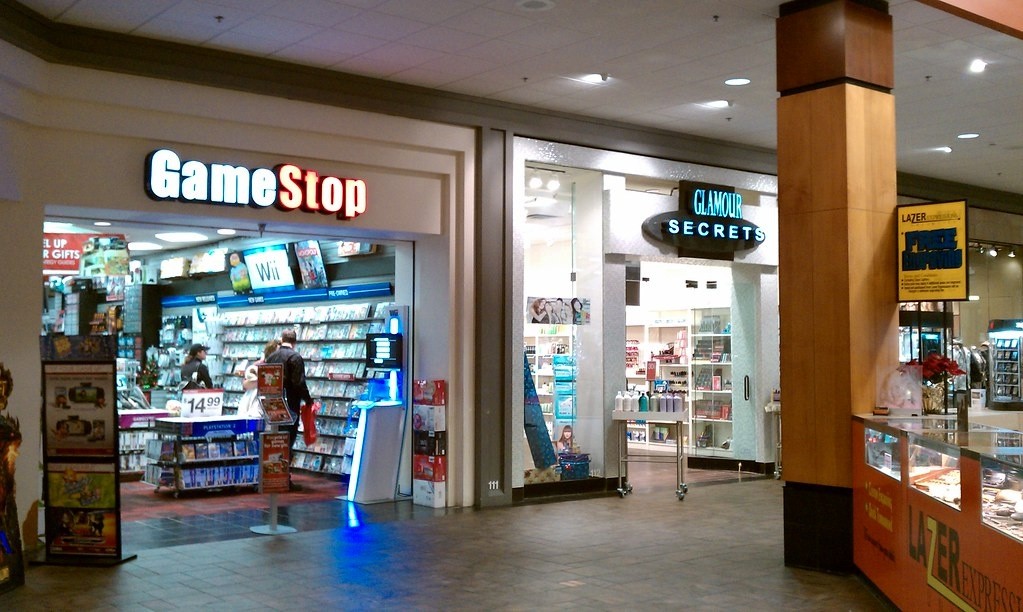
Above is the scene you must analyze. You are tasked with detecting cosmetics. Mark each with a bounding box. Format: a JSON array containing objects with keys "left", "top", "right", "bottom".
[{"left": 526, "top": 319, "right": 731, "bottom": 440}]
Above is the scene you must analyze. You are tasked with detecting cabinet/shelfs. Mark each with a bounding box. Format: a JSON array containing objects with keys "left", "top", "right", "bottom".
[
  {"left": 40, "top": 239, "right": 397, "bottom": 496},
  {"left": 523, "top": 308, "right": 731, "bottom": 472}
]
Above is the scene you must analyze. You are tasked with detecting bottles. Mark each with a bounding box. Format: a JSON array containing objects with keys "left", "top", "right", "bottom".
[{"left": 615, "top": 390, "right": 683, "bottom": 413}]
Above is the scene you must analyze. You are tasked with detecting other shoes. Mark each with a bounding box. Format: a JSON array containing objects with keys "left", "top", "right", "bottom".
[{"left": 289, "top": 481, "right": 302, "bottom": 492}]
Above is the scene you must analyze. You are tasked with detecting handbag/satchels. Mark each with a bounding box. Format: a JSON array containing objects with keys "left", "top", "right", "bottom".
[
  {"left": 178, "top": 379, "right": 207, "bottom": 392},
  {"left": 301, "top": 402, "right": 321, "bottom": 445}
]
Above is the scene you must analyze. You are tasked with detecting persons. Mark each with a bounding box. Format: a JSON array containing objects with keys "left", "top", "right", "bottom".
[
  {"left": 530, "top": 298, "right": 584, "bottom": 324},
  {"left": 182, "top": 344, "right": 212, "bottom": 389},
  {"left": 267, "top": 331, "right": 314, "bottom": 490},
  {"left": 238, "top": 340, "right": 281, "bottom": 415},
  {"left": 557, "top": 425, "right": 572, "bottom": 450}
]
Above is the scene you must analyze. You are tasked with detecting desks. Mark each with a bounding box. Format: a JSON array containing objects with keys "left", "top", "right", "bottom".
[
  {"left": 611, "top": 409, "right": 689, "bottom": 499},
  {"left": 765, "top": 403, "right": 782, "bottom": 480}
]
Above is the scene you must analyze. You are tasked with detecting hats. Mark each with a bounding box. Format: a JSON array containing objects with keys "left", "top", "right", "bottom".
[{"left": 189, "top": 344, "right": 210, "bottom": 355}]
